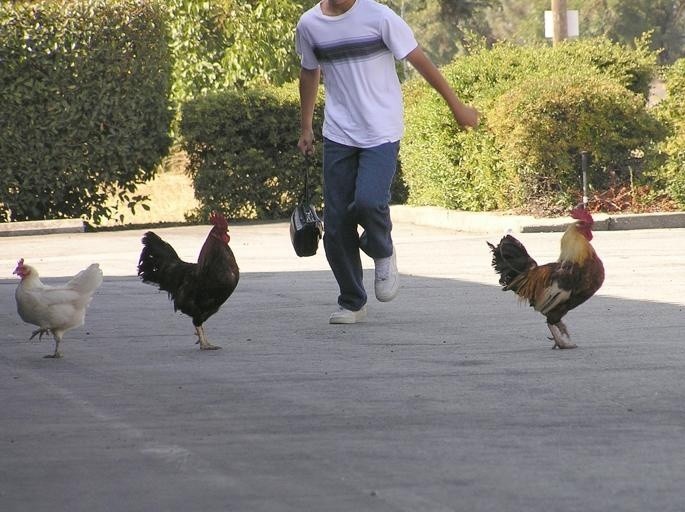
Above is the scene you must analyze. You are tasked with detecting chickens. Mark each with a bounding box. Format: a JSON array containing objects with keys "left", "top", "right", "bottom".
[
  {"left": 486, "top": 207, "right": 605, "bottom": 349},
  {"left": 12, "top": 257, "right": 104, "bottom": 359},
  {"left": 136, "top": 212, "right": 239, "bottom": 351}
]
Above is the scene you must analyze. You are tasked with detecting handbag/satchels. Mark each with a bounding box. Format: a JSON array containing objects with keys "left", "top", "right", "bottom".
[{"left": 290, "top": 150, "right": 323, "bottom": 257}]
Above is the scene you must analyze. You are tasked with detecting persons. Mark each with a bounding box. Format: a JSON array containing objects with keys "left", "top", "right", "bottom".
[{"left": 293, "top": 0, "right": 483, "bottom": 324}]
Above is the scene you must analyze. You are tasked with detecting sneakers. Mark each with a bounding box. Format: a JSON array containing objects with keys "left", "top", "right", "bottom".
[
  {"left": 374, "top": 246, "right": 400, "bottom": 302},
  {"left": 330, "top": 303, "right": 367, "bottom": 324}
]
